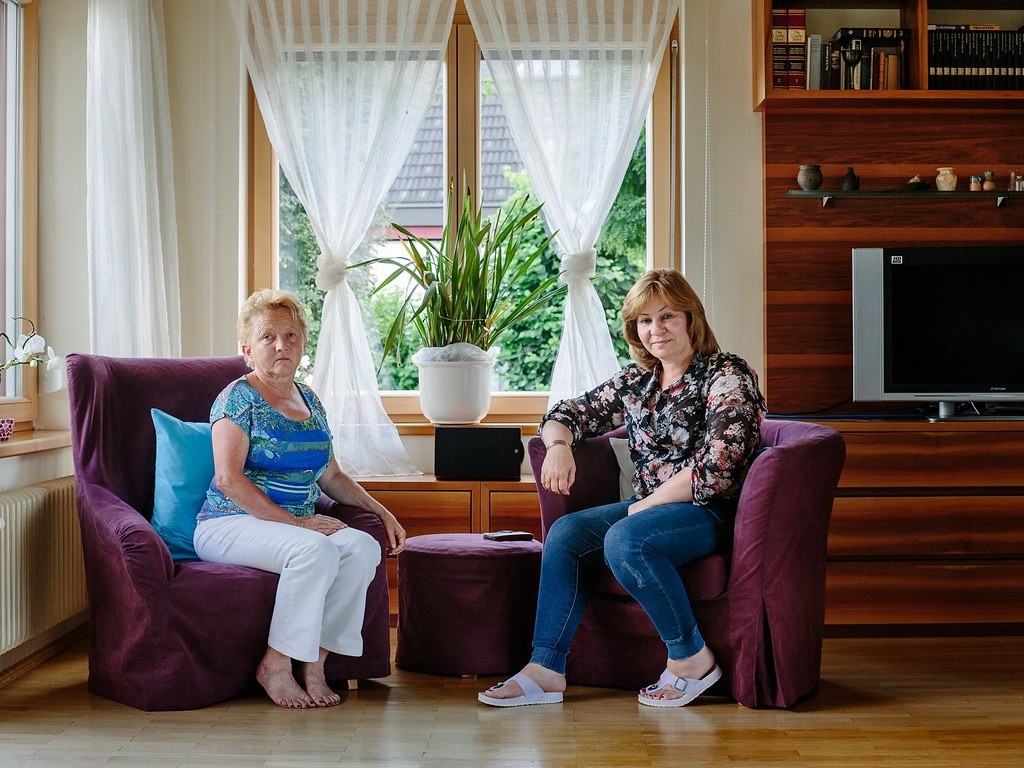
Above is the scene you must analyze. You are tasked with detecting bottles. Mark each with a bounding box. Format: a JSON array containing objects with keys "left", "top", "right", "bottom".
[
  {"left": 1015, "top": 176, "right": 1023, "bottom": 191},
  {"left": 842, "top": 167, "right": 859, "bottom": 191},
  {"left": 1007, "top": 172, "right": 1016, "bottom": 191},
  {"left": 936, "top": 167, "right": 957, "bottom": 191},
  {"left": 969, "top": 175, "right": 983, "bottom": 191},
  {"left": 797, "top": 165, "right": 823, "bottom": 191},
  {"left": 983, "top": 171, "right": 995, "bottom": 190}
]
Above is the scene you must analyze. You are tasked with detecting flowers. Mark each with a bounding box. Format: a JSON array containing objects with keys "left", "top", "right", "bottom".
[{"left": 0, "top": 316, "right": 65, "bottom": 385}]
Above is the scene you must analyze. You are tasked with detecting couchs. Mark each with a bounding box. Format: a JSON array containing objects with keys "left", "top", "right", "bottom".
[
  {"left": 528, "top": 419, "right": 846, "bottom": 711},
  {"left": 66, "top": 351, "right": 394, "bottom": 714}
]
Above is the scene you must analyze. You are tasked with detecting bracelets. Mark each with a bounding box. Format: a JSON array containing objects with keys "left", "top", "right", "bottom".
[{"left": 545, "top": 440, "right": 572, "bottom": 449}]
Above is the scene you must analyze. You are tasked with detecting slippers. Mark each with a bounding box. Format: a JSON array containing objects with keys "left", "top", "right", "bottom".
[
  {"left": 477, "top": 671, "right": 564, "bottom": 707},
  {"left": 637, "top": 663, "right": 723, "bottom": 708}
]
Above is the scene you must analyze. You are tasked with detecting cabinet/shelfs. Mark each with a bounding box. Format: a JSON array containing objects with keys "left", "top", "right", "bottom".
[{"left": 750, "top": 0, "right": 1024, "bottom": 112}]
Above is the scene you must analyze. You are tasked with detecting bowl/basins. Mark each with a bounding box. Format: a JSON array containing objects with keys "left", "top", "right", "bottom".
[{"left": 0, "top": 418, "right": 15, "bottom": 442}]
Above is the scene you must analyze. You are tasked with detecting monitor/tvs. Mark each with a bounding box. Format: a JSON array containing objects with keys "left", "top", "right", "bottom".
[{"left": 851, "top": 247, "right": 1024, "bottom": 418}]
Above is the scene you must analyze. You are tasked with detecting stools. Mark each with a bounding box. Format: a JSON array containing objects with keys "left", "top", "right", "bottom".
[{"left": 392, "top": 532, "right": 544, "bottom": 675}]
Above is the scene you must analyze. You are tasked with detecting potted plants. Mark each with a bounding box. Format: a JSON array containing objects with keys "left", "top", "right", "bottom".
[{"left": 342, "top": 167, "right": 601, "bottom": 423}]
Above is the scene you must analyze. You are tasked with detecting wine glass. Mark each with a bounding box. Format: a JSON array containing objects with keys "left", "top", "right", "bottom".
[{"left": 841, "top": 49, "right": 863, "bottom": 90}]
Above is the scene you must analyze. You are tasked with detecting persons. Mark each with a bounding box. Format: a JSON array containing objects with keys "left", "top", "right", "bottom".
[
  {"left": 192, "top": 287, "right": 408, "bottom": 708},
  {"left": 475, "top": 269, "right": 772, "bottom": 708}
]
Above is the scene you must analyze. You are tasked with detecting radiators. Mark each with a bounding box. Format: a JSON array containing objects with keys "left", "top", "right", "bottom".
[{"left": 0, "top": 474, "right": 89, "bottom": 656}]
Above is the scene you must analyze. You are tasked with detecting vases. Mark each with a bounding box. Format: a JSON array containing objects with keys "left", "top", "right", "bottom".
[
  {"left": 983, "top": 171, "right": 997, "bottom": 192},
  {"left": 0, "top": 417, "right": 15, "bottom": 442},
  {"left": 796, "top": 165, "right": 823, "bottom": 190},
  {"left": 841, "top": 166, "right": 859, "bottom": 191},
  {"left": 934, "top": 167, "right": 957, "bottom": 192},
  {"left": 969, "top": 176, "right": 982, "bottom": 191}
]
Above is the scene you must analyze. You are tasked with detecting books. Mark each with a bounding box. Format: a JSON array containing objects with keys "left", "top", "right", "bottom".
[{"left": 769, "top": 5, "right": 1024, "bottom": 92}]
